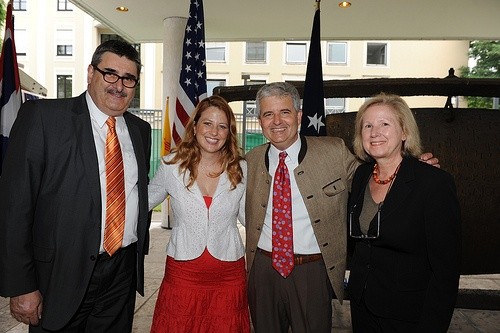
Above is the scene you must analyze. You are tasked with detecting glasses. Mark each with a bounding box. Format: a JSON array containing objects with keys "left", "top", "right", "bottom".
[
  {"left": 93, "top": 66, "right": 139, "bottom": 88},
  {"left": 349, "top": 200, "right": 383, "bottom": 238}
]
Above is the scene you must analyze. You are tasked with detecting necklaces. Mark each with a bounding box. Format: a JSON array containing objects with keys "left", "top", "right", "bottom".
[{"left": 372, "top": 161, "right": 404, "bottom": 185}]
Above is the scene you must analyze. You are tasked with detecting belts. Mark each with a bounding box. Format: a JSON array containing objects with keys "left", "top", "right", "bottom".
[{"left": 255, "top": 247, "right": 322, "bottom": 265}]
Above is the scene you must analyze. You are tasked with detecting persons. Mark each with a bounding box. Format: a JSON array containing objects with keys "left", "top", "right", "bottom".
[
  {"left": 148, "top": 95, "right": 252, "bottom": 332},
  {"left": 241, "top": 82, "right": 441, "bottom": 332},
  {"left": 1, "top": 38, "right": 152, "bottom": 333},
  {"left": 344, "top": 93, "right": 464, "bottom": 333}
]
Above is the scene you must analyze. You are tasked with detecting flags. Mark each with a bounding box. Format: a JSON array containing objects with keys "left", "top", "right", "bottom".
[
  {"left": 171, "top": 0, "right": 208, "bottom": 154},
  {"left": 0, "top": 0, "right": 24, "bottom": 141},
  {"left": 160, "top": 95, "right": 176, "bottom": 229},
  {"left": 299, "top": 1, "right": 327, "bottom": 137}
]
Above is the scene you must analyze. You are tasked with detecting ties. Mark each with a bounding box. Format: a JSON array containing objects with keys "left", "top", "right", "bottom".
[
  {"left": 104, "top": 115, "right": 126, "bottom": 256},
  {"left": 272, "top": 150, "right": 295, "bottom": 278}
]
